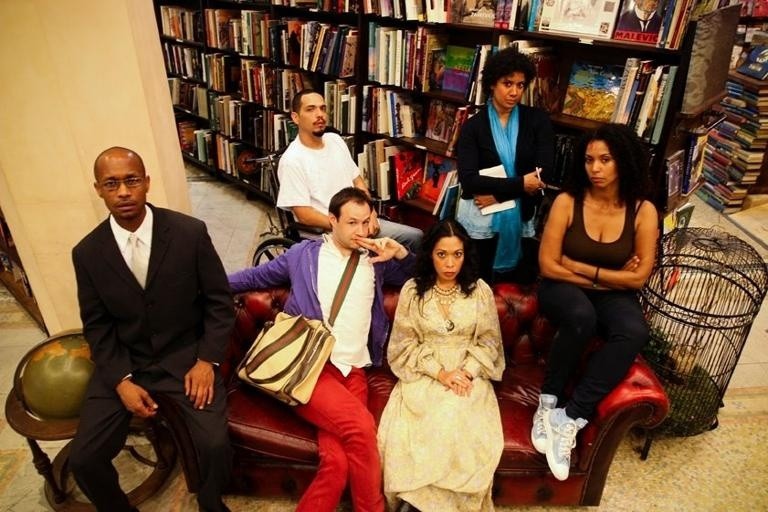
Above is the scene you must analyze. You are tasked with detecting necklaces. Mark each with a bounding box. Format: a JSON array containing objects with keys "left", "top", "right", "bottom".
[{"left": 431, "top": 283, "right": 459, "bottom": 305}]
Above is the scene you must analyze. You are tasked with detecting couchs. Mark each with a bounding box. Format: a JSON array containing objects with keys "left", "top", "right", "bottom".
[{"left": 158, "top": 286, "right": 670, "bottom": 505}]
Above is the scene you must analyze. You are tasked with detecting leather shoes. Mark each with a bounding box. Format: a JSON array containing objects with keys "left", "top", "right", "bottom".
[{"left": 197, "top": 497, "right": 232, "bottom": 512}]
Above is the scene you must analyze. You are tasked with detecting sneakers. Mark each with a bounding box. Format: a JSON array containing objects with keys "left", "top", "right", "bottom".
[{"left": 531, "top": 394, "right": 589, "bottom": 482}]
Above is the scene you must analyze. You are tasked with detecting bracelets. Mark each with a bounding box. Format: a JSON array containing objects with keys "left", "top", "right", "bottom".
[{"left": 594, "top": 267, "right": 601, "bottom": 283}]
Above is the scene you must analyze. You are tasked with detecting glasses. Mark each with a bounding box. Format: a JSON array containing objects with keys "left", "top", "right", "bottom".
[{"left": 100, "top": 178, "right": 144, "bottom": 191}]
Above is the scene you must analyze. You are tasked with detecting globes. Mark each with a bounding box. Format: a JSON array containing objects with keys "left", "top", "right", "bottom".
[{"left": 5, "top": 327, "right": 178, "bottom": 511}]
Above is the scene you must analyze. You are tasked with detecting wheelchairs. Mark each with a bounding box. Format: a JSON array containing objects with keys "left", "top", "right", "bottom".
[{"left": 243, "top": 138, "right": 325, "bottom": 267}]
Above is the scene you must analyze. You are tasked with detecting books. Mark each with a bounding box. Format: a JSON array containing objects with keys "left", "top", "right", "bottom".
[{"left": 692, "top": 0, "right": 768, "bottom": 213}]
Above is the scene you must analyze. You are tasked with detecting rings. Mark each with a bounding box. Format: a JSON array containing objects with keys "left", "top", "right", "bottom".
[
  {"left": 376, "top": 217, "right": 505, "bottom": 512},
  {"left": 456, "top": 375, "right": 462, "bottom": 380}
]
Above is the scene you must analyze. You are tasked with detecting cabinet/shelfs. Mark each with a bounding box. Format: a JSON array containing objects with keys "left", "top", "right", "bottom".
[
  {"left": 153, "top": 1, "right": 742, "bottom": 271},
  {"left": 695, "top": 2, "right": 768, "bottom": 213},
  {"left": 1, "top": 217, "right": 51, "bottom": 339}
]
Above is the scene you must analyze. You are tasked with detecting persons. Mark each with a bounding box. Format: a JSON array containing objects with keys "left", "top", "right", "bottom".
[
  {"left": 454, "top": 47, "right": 546, "bottom": 286},
  {"left": 276, "top": 89, "right": 422, "bottom": 287},
  {"left": 226, "top": 187, "right": 417, "bottom": 511},
  {"left": 159, "top": 1, "right": 708, "bottom": 235},
  {"left": 532, "top": 122, "right": 657, "bottom": 482},
  {"left": 66, "top": 147, "right": 233, "bottom": 512}
]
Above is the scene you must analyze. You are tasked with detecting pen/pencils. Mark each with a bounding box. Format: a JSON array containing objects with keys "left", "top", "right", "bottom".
[{"left": 535, "top": 167, "right": 545, "bottom": 197}]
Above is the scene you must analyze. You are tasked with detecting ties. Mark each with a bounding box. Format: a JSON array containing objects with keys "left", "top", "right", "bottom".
[{"left": 129, "top": 236, "right": 145, "bottom": 290}]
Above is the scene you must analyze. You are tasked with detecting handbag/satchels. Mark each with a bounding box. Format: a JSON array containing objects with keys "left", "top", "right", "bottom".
[{"left": 235, "top": 312, "right": 336, "bottom": 406}]
